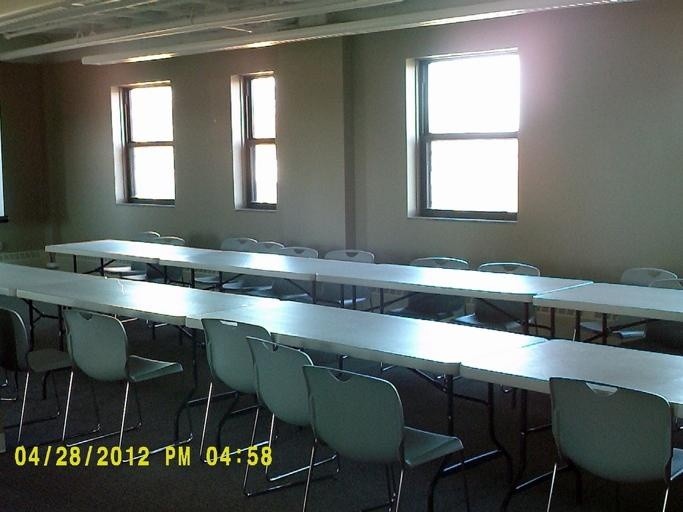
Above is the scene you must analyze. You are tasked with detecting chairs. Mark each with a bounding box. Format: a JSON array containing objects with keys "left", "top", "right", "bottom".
[
  {"left": 0, "top": 228, "right": 539, "bottom": 511},
  {"left": 573, "top": 267, "right": 678, "bottom": 346},
  {"left": 546, "top": 377, "right": 683, "bottom": 511}
]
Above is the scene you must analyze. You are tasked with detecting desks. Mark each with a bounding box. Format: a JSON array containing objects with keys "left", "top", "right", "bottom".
[
  {"left": 460, "top": 340, "right": 682, "bottom": 511},
  {"left": 533, "top": 282, "right": 682, "bottom": 357}
]
